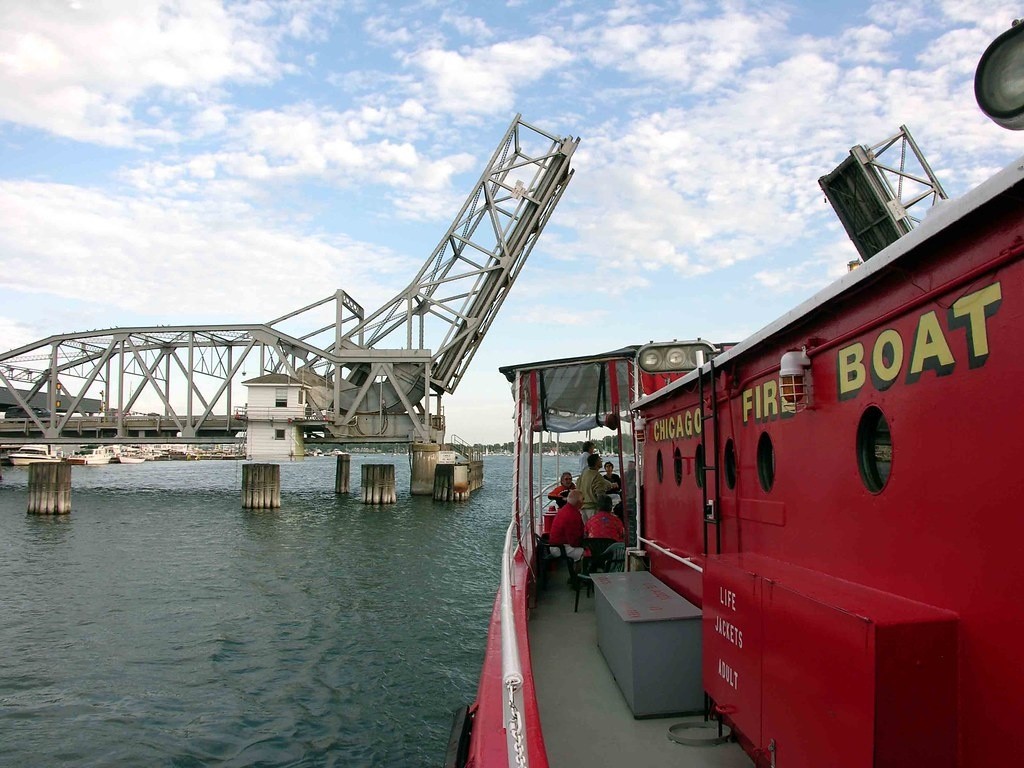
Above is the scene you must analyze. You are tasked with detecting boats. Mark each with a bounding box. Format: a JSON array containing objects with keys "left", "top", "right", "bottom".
[
  {"left": 118, "top": 447, "right": 146, "bottom": 464},
  {"left": 7, "top": 445, "right": 66, "bottom": 465},
  {"left": 445, "top": 12, "right": 1023, "bottom": 768},
  {"left": 331, "top": 448, "right": 352, "bottom": 456},
  {"left": 67, "top": 447, "right": 112, "bottom": 465}
]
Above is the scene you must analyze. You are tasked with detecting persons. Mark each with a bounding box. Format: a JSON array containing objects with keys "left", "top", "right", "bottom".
[
  {"left": 579, "top": 442, "right": 595, "bottom": 470},
  {"left": 603, "top": 462, "right": 622, "bottom": 495},
  {"left": 586, "top": 496, "right": 625, "bottom": 545},
  {"left": 576, "top": 454, "right": 619, "bottom": 524},
  {"left": 549, "top": 472, "right": 575, "bottom": 500},
  {"left": 550, "top": 490, "right": 591, "bottom": 571}
]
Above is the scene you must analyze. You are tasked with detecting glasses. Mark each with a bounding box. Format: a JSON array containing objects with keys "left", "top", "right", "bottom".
[
  {"left": 582, "top": 497, "right": 584, "bottom": 500},
  {"left": 563, "top": 478, "right": 572, "bottom": 481}
]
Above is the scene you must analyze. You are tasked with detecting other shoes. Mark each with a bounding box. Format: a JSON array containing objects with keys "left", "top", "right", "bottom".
[{"left": 566, "top": 576, "right": 587, "bottom": 587}]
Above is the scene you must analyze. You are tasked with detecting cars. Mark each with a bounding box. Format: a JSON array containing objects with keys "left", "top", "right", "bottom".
[{"left": 5, "top": 406, "right": 64, "bottom": 423}]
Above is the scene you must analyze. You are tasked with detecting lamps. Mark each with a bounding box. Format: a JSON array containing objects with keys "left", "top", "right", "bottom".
[
  {"left": 779, "top": 351, "right": 811, "bottom": 414},
  {"left": 974, "top": 18, "right": 1024, "bottom": 131},
  {"left": 636, "top": 338, "right": 716, "bottom": 374}
]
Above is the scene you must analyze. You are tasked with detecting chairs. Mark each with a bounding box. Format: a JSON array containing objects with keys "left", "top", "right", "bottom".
[
  {"left": 573, "top": 538, "right": 625, "bottom": 613},
  {"left": 534, "top": 534, "right": 575, "bottom": 589}
]
